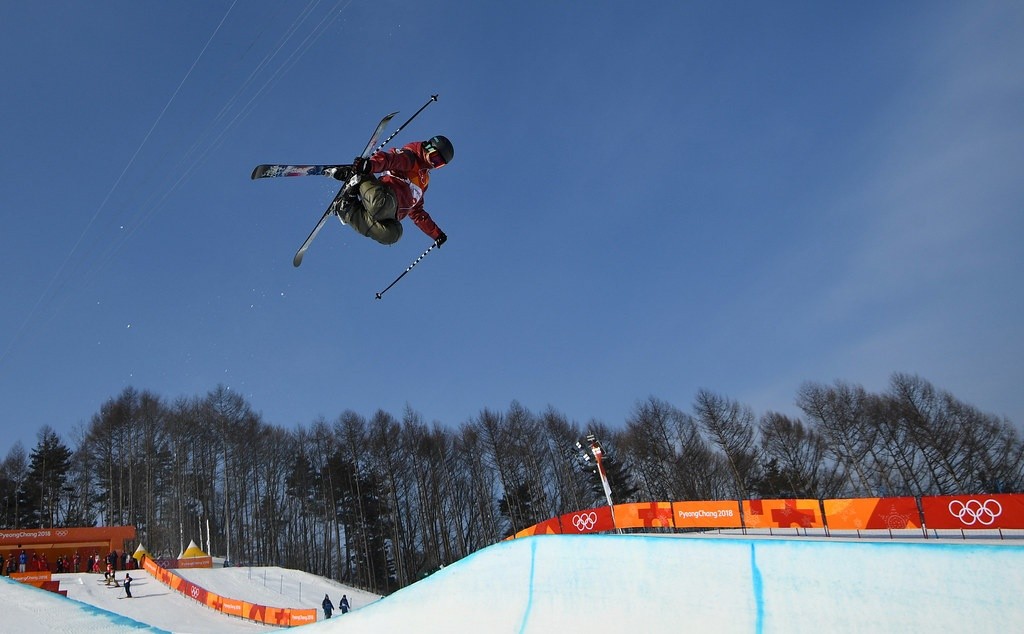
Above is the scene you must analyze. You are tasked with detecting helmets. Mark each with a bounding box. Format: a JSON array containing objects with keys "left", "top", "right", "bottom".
[{"left": 428, "top": 135, "right": 454, "bottom": 164}]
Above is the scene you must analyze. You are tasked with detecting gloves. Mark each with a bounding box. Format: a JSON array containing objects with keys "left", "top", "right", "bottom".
[
  {"left": 435, "top": 233, "right": 447, "bottom": 249},
  {"left": 351, "top": 157, "right": 372, "bottom": 175}
]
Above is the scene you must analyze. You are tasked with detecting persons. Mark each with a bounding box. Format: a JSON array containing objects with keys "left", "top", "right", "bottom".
[
  {"left": 18, "top": 549, "right": 28, "bottom": 573},
  {"left": 332, "top": 135, "right": 455, "bottom": 243},
  {"left": 5, "top": 553, "right": 16, "bottom": 576},
  {"left": 105, "top": 550, "right": 138, "bottom": 570},
  {"left": 322, "top": 594, "right": 335, "bottom": 619},
  {"left": 339, "top": 594, "right": 350, "bottom": 614},
  {"left": 87, "top": 550, "right": 102, "bottom": 574},
  {"left": 123, "top": 573, "right": 132, "bottom": 598},
  {"left": 31, "top": 552, "right": 48, "bottom": 571},
  {"left": 0, "top": 554, "right": 4, "bottom": 575},
  {"left": 104, "top": 564, "right": 120, "bottom": 588},
  {"left": 73, "top": 550, "right": 80, "bottom": 573},
  {"left": 57, "top": 555, "right": 70, "bottom": 573}
]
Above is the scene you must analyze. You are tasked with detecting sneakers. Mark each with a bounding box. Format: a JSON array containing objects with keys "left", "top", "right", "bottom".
[
  {"left": 332, "top": 195, "right": 358, "bottom": 217},
  {"left": 332, "top": 167, "right": 353, "bottom": 182}
]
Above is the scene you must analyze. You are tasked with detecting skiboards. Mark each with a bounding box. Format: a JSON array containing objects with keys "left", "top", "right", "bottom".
[
  {"left": 250, "top": 111, "right": 401, "bottom": 268},
  {"left": 97, "top": 584, "right": 125, "bottom": 589}
]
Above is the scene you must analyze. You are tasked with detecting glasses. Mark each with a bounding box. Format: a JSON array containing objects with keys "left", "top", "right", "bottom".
[{"left": 427, "top": 150, "right": 446, "bottom": 169}]
